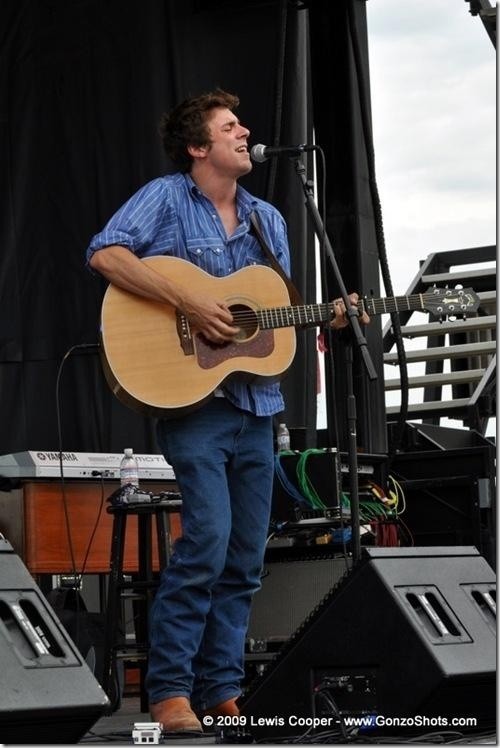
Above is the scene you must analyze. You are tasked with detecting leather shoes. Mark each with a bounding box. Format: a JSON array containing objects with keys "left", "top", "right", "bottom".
[
  {"left": 146, "top": 695, "right": 203, "bottom": 735},
  {"left": 196, "top": 694, "right": 242, "bottom": 725}
]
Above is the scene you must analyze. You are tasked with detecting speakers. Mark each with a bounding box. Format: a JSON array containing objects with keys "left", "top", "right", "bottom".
[
  {"left": 246, "top": 558, "right": 352, "bottom": 651},
  {"left": 0, "top": 533, "right": 111, "bottom": 746},
  {"left": 216, "top": 545, "right": 498, "bottom": 742}
]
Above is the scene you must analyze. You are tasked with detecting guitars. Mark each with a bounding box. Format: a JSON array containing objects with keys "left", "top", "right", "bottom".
[{"left": 100, "top": 256, "right": 482, "bottom": 420}]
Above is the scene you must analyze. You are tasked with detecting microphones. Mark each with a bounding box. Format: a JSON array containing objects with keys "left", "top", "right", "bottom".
[{"left": 250, "top": 142, "right": 312, "bottom": 163}]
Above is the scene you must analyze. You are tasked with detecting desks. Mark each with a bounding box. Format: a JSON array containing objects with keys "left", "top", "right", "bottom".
[{"left": 0, "top": 479, "right": 194, "bottom": 711}]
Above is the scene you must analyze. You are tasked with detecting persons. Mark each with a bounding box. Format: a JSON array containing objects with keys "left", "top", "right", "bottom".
[{"left": 85, "top": 86, "right": 370, "bottom": 737}]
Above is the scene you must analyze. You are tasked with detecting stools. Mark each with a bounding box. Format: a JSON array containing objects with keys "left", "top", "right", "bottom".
[{"left": 91, "top": 499, "right": 211, "bottom": 714}]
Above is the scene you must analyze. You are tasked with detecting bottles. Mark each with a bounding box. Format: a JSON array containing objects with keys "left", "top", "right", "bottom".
[
  {"left": 277, "top": 423, "right": 289, "bottom": 451},
  {"left": 119, "top": 447, "right": 138, "bottom": 492}
]
